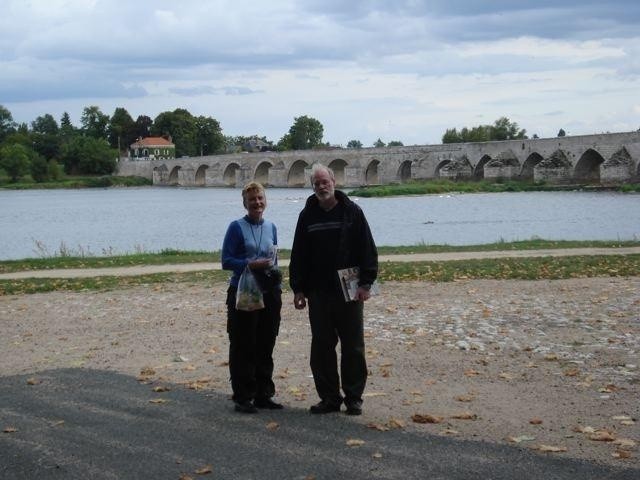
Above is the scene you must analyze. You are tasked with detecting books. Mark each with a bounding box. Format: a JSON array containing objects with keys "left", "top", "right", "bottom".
[{"left": 335, "top": 265, "right": 380, "bottom": 303}]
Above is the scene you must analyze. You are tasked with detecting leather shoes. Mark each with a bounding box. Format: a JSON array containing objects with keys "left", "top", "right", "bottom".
[
  {"left": 234, "top": 398, "right": 283, "bottom": 413},
  {"left": 311, "top": 400, "right": 340, "bottom": 414},
  {"left": 345, "top": 399, "right": 362, "bottom": 415}
]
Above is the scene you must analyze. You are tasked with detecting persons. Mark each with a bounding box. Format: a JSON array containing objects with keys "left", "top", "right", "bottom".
[
  {"left": 220, "top": 181, "right": 284, "bottom": 414},
  {"left": 288, "top": 162, "right": 378, "bottom": 414}
]
